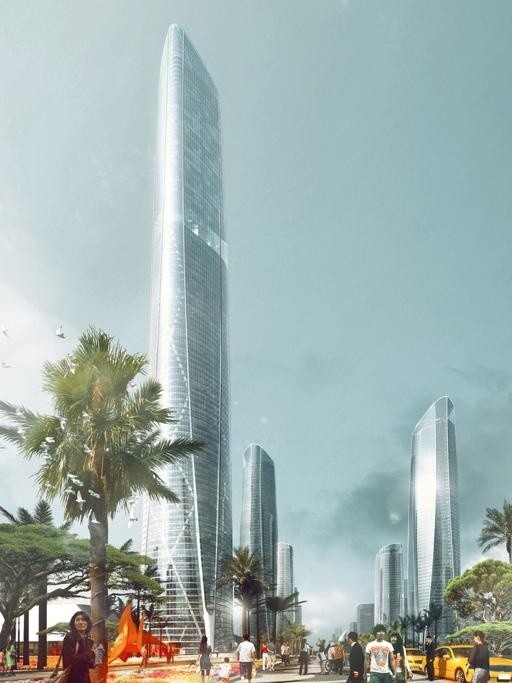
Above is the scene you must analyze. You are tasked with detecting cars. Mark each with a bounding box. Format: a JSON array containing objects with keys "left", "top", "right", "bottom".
[
  {"left": 421, "top": 642, "right": 512, "bottom": 683},
  {"left": 404, "top": 647, "right": 426, "bottom": 673}
]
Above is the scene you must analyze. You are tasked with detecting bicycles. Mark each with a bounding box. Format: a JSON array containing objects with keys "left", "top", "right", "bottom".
[{"left": 324, "top": 660, "right": 335, "bottom": 672}]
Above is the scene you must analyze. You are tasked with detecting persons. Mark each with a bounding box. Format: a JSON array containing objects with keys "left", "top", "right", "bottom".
[
  {"left": 62, "top": 611, "right": 98, "bottom": 682},
  {"left": 236, "top": 633, "right": 257, "bottom": 683},
  {"left": 6, "top": 640, "right": 16, "bottom": 676},
  {"left": 197, "top": 636, "right": 214, "bottom": 683},
  {"left": 465, "top": 630, "right": 489, "bottom": 682},
  {"left": 0, "top": 648, "right": 5, "bottom": 673},
  {"left": 218, "top": 658, "right": 231, "bottom": 683},
  {"left": 262, "top": 625, "right": 411, "bottom": 683},
  {"left": 425, "top": 634, "right": 435, "bottom": 679}
]
